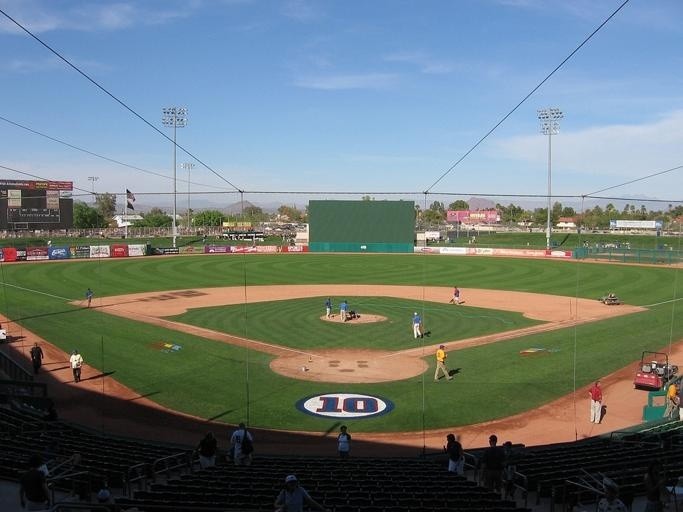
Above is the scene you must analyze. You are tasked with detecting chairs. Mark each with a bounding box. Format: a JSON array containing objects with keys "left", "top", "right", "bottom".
[{"left": 0, "top": 405, "right": 682, "bottom": 512}]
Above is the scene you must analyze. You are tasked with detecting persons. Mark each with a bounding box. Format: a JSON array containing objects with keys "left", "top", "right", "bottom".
[
  {"left": 325, "top": 298, "right": 356, "bottom": 322},
  {"left": 644, "top": 458, "right": 662, "bottom": 510},
  {"left": 412, "top": 311, "right": 424, "bottom": 339},
  {"left": 454, "top": 287, "right": 463, "bottom": 305},
  {"left": 434, "top": 344, "right": 453, "bottom": 381},
  {"left": 93, "top": 489, "right": 138, "bottom": 511},
  {"left": 30, "top": 342, "right": 43, "bottom": 373},
  {"left": 444, "top": 435, "right": 465, "bottom": 473},
  {"left": 597, "top": 483, "right": 627, "bottom": 510},
  {"left": 196, "top": 432, "right": 217, "bottom": 468},
  {"left": 230, "top": 423, "right": 253, "bottom": 466},
  {"left": 86, "top": 287, "right": 93, "bottom": 307},
  {"left": 336, "top": 426, "right": 351, "bottom": 457},
  {"left": 588, "top": 382, "right": 603, "bottom": 425},
  {"left": 21, "top": 398, "right": 57, "bottom": 421},
  {"left": 274, "top": 475, "right": 325, "bottom": 511},
  {"left": 70, "top": 349, "right": 83, "bottom": 382},
  {"left": 663, "top": 380, "right": 681, "bottom": 419},
  {"left": 20, "top": 455, "right": 50, "bottom": 511},
  {"left": 477, "top": 435, "right": 513, "bottom": 496}
]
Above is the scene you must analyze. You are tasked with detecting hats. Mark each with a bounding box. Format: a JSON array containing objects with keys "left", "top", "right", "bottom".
[{"left": 285, "top": 475, "right": 297, "bottom": 483}]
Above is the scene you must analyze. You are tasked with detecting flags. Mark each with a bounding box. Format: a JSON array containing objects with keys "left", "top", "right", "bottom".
[
  {"left": 127, "top": 190, "right": 134, "bottom": 200},
  {"left": 127, "top": 200, "right": 136, "bottom": 212}
]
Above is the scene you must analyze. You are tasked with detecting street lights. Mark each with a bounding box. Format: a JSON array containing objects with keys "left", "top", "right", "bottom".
[
  {"left": 178, "top": 160, "right": 198, "bottom": 234},
  {"left": 536, "top": 106, "right": 567, "bottom": 249},
  {"left": 162, "top": 105, "right": 190, "bottom": 250},
  {"left": 88, "top": 173, "right": 101, "bottom": 195}
]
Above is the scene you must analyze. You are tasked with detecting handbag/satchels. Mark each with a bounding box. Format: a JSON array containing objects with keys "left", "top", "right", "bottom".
[{"left": 241, "top": 438, "right": 253, "bottom": 454}]
